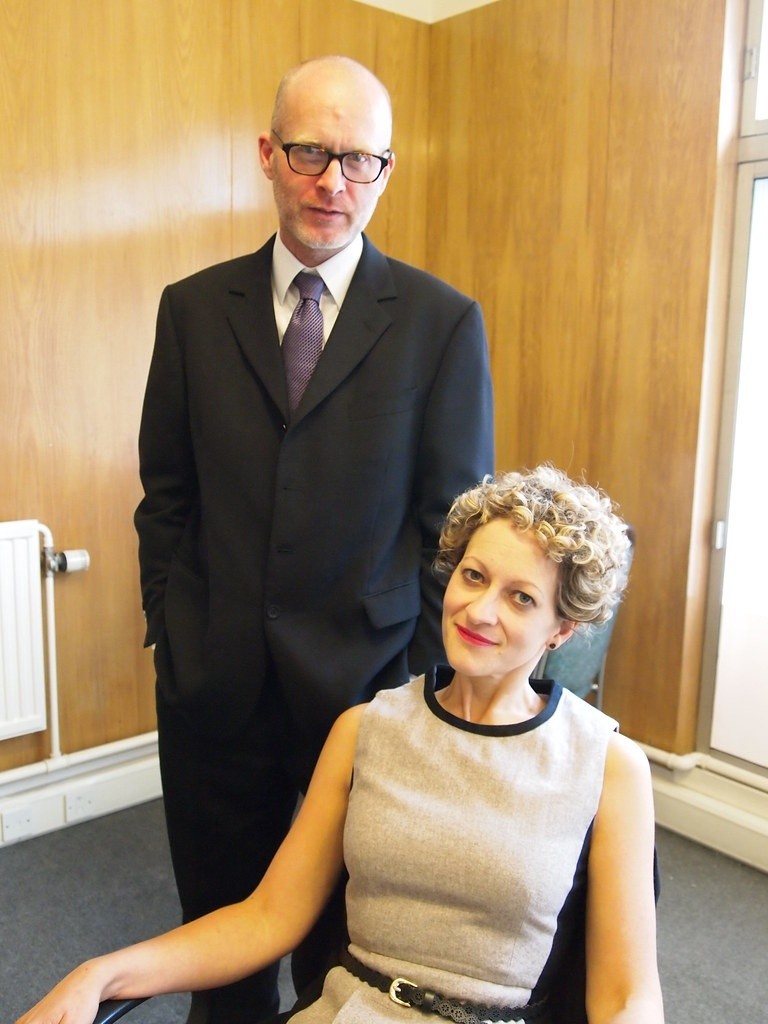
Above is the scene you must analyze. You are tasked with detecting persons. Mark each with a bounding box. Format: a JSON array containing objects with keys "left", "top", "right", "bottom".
[
  {"left": 21, "top": 465, "right": 664, "bottom": 1024},
  {"left": 134, "top": 53, "right": 493, "bottom": 1024}
]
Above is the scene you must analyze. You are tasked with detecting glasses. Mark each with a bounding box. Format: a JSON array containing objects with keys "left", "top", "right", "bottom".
[{"left": 272, "top": 128, "right": 393, "bottom": 184}]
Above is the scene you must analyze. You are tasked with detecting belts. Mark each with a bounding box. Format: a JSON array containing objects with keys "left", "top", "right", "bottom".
[{"left": 339, "top": 946, "right": 551, "bottom": 1024}]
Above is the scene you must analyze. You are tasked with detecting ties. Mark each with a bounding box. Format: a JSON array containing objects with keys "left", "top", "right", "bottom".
[{"left": 281, "top": 271, "right": 325, "bottom": 421}]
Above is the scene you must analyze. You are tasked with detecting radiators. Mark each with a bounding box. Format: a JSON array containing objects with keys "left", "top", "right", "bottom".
[{"left": 0, "top": 519, "right": 89, "bottom": 741}]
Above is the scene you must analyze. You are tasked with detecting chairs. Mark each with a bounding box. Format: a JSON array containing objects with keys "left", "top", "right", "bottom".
[{"left": 531, "top": 527, "right": 637, "bottom": 713}]
